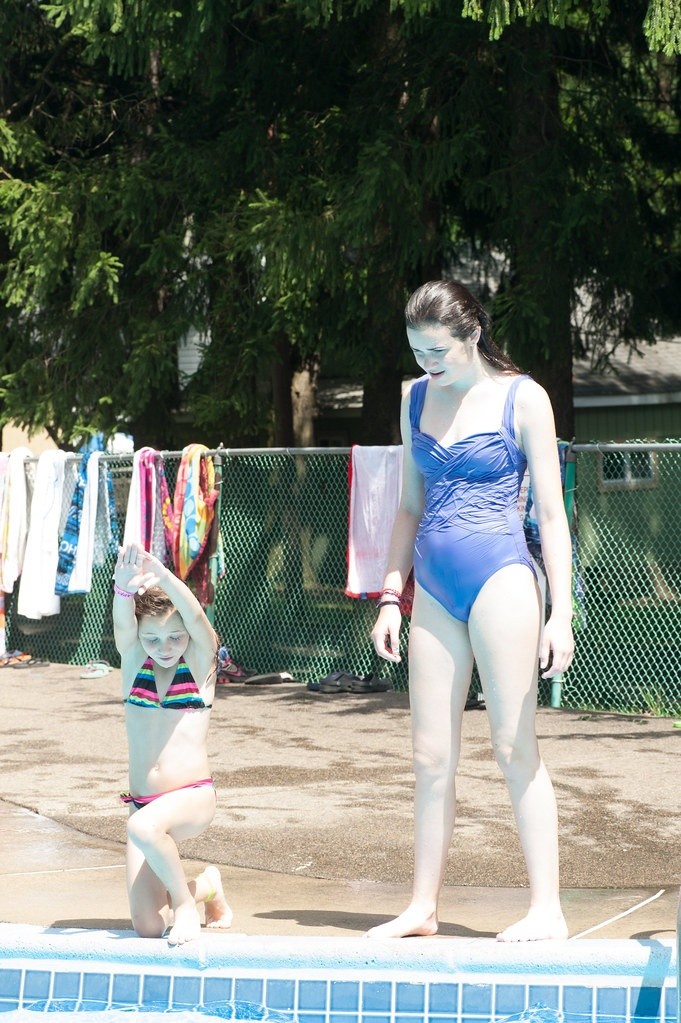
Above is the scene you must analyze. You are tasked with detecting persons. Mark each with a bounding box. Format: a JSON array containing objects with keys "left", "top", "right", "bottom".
[
  {"left": 112, "top": 544, "right": 234, "bottom": 946},
  {"left": 366, "top": 280, "right": 574, "bottom": 942}
]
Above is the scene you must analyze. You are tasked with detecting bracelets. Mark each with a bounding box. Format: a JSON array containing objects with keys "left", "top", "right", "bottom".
[
  {"left": 114, "top": 584, "right": 134, "bottom": 598},
  {"left": 379, "top": 588, "right": 401, "bottom": 599},
  {"left": 376, "top": 600, "right": 400, "bottom": 610}
]
